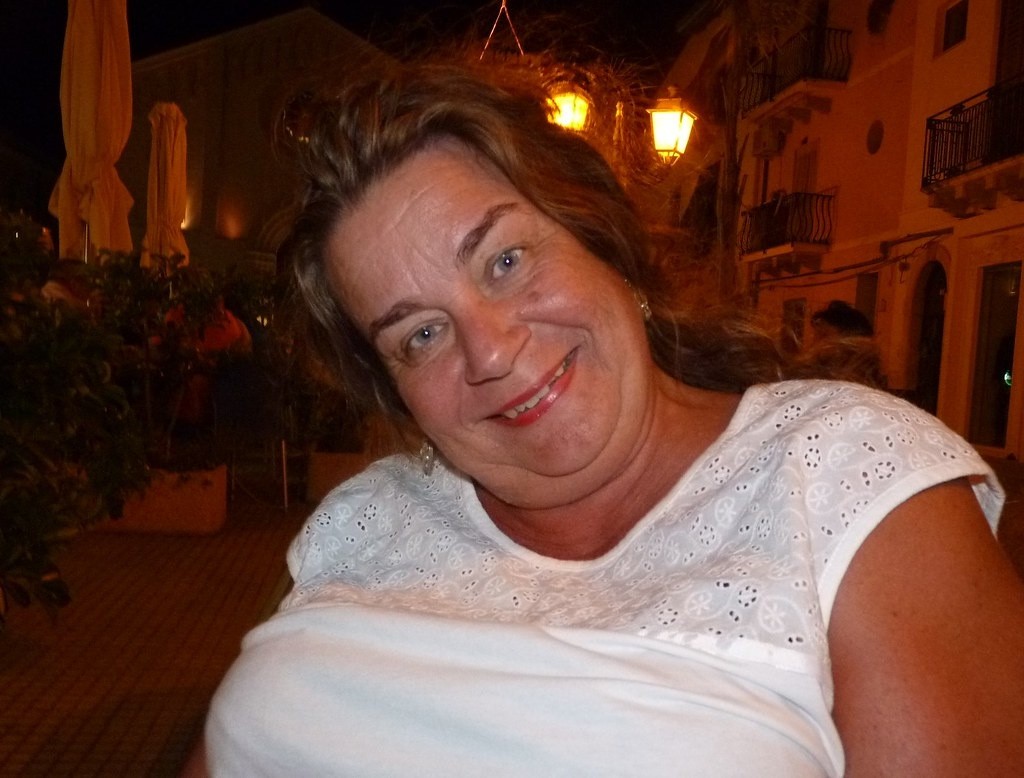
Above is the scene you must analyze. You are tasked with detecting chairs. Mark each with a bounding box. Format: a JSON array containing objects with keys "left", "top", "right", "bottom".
[{"left": 165, "top": 351, "right": 293, "bottom": 516}]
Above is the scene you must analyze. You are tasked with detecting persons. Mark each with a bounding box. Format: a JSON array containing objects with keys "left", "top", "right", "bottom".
[
  {"left": 810, "top": 302, "right": 880, "bottom": 388},
  {"left": 187, "top": 54, "right": 1024, "bottom": 778},
  {"left": 165, "top": 281, "right": 256, "bottom": 427}
]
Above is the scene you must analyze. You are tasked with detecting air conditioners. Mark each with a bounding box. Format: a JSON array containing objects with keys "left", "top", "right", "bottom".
[{"left": 750, "top": 122, "right": 784, "bottom": 160}]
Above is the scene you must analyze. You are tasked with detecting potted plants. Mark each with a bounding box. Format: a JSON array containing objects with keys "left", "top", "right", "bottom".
[
  {"left": 0, "top": 206, "right": 252, "bottom": 631},
  {"left": 303, "top": 381, "right": 372, "bottom": 503}
]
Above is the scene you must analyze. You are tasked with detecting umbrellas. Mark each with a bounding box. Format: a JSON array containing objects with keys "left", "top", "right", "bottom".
[
  {"left": 49, "top": 0, "right": 135, "bottom": 272},
  {"left": 146, "top": 105, "right": 192, "bottom": 278}
]
